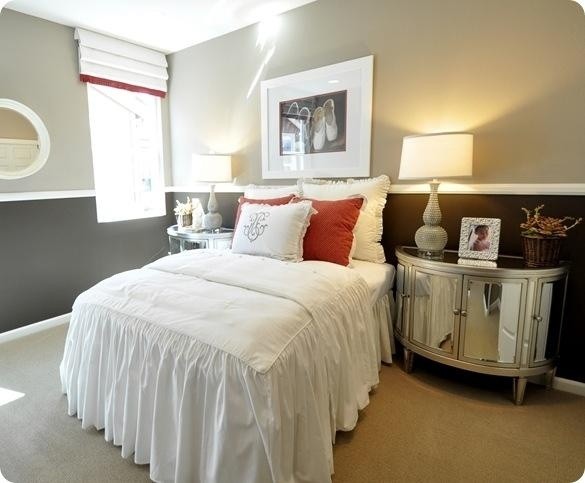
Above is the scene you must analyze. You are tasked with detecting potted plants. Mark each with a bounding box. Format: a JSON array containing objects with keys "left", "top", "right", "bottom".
[
  {"left": 519, "top": 201, "right": 582, "bottom": 267},
  {"left": 174, "top": 196, "right": 192, "bottom": 227}
]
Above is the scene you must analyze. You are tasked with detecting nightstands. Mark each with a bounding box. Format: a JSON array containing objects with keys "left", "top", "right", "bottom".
[
  {"left": 392, "top": 246, "right": 569, "bottom": 406},
  {"left": 196, "top": 152, "right": 236, "bottom": 230},
  {"left": 167, "top": 223, "right": 235, "bottom": 255}
]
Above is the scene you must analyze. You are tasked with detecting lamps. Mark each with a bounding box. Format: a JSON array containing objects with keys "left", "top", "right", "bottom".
[{"left": 398, "top": 131, "right": 473, "bottom": 259}]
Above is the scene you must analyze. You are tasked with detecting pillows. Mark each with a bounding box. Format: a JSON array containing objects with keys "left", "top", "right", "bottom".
[{"left": 230, "top": 173, "right": 392, "bottom": 268}]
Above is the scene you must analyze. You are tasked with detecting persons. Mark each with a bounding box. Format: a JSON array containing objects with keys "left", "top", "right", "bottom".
[{"left": 470, "top": 225, "right": 490, "bottom": 251}]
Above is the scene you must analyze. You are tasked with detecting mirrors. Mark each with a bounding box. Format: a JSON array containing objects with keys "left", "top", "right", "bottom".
[{"left": 0, "top": 97, "right": 51, "bottom": 179}]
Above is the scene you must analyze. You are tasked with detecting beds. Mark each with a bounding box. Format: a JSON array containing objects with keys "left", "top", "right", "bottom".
[{"left": 59, "top": 245, "right": 397, "bottom": 481}]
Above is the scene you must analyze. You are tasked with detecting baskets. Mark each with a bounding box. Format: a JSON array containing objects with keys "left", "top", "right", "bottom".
[{"left": 521, "top": 234, "right": 567, "bottom": 269}]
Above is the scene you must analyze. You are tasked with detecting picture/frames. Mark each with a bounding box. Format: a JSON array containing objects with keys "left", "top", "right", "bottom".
[
  {"left": 458, "top": 216, "right": 501, "bottom": 261},
  {"left": 260, "top": 54, "right": 375, "bottom": 178}
]
